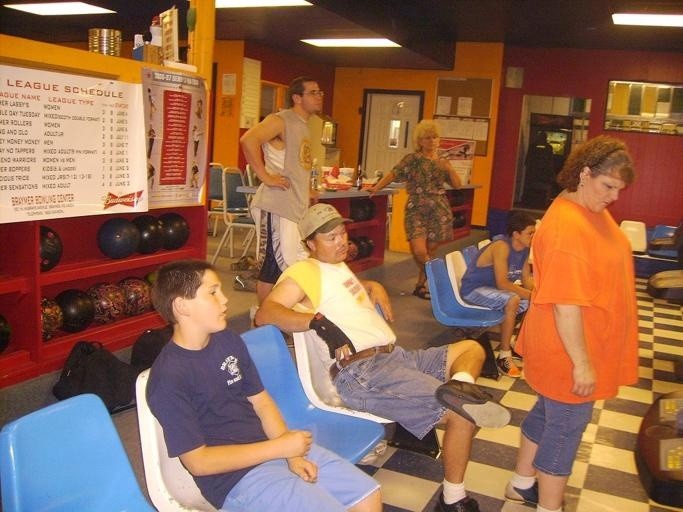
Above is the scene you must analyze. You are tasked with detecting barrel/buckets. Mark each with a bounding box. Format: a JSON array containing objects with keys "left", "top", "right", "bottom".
[
  {"left": 321, "top": 166, "right": 334, "bottom": 178},
  {"left": 449, "top": 159, "right": 474, "bottom": 187},
  {"left": 340, "top": 167, "right": 355, "bottom": 178}
]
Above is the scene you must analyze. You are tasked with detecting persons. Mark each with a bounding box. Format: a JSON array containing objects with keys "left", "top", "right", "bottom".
[
  {"left": 146, "top": 90, "right": 203, "bottom": 189},
  {"left": 146, "top": 258, "right": 383, "bottom": 511},
  {"left": 240, "top": 75, "right": 323, "bottom": 308},
  {"left": 255, "top": 203, "right": 512, "bottom": 511},
  {"left": 460, "top": 212, "right": 536, "bottom": 378},
  {"left": 361, "top": 119, "right": 460, "bottom": 296},
  {"left": 504, "top": 136, "right": 639, "bottom": 512}
]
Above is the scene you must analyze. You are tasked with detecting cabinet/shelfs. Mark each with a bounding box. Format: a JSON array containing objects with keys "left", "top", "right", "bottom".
[
  {"left": 387, "top": 181, "right": 482, "bottom": 254},
  {"left": 236, "top": 185, "right": 398, "bottom": 273},
  {"left": 0, "top": 207, "right": 209, "bottom": 390}
]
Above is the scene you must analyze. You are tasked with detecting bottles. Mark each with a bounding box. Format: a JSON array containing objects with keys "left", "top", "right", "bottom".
[
  {"left": 355, "top": 164, "right": 363, "bottom": 190},
  {"left": 311, "top": 159, "right": 321, "bottom": 191}
]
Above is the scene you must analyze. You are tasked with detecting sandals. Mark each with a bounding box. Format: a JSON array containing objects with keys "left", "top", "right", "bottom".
[{"left": 413, "top": 286, "right": 432, "bottom": 300}]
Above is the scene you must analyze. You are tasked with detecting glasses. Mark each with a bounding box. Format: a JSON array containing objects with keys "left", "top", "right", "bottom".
[{"left": 298, "top": 90, "right": 325, "bottom": 97}]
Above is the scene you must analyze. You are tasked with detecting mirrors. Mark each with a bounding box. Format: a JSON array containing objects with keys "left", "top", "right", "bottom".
[{"left": 603, "top": 79, "right": 683, "bottom": 135}]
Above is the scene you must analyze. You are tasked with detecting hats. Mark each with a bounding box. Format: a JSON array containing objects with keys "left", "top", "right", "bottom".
[{"left": 299, "top": 203, "right": 353, "bottom": 241}]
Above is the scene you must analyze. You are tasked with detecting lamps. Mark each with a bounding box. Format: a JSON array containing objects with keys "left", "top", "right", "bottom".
[
  {"left": 3, "top": 1, "right": 119, "bottom": 16},
  {"left": 299, "top": 31, "right": 402, "bottom": 48}
]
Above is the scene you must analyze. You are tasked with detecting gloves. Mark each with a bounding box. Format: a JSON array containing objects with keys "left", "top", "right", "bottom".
[
  {"left": 308, "top": 312, "right": 356, "bottom": 359},
  {"left": 328, "top": 342, "right": 394, "bottom": 378}
]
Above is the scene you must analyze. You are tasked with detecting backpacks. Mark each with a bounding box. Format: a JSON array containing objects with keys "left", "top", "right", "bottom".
[{"left": 130, "top": 328, "right": 173, "bottom": 371}]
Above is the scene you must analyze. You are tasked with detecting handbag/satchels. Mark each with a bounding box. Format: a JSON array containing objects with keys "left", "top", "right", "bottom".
[{"left": 54, "top": 340, "right": 137, "bottom": 410}]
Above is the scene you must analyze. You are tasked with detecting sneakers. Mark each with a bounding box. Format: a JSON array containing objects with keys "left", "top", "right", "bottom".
[
  {"left": 504, "top": 479, "right": 538, "bottom": 506},
  {"left": 433, "top": 491, "right": 482, "bottom": 511},
  {"left": 434, "top": 380, "right": 512, "bottom": 428},
  {"left": 495, "top": 345, "right": 522, "bottom": 378}
]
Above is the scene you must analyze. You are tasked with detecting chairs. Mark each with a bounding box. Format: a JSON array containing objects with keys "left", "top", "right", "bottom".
[
  {"left": 207, "top": 163, "right": 224, "bottom": 236},
  {"left": 619, "top": 220, "right": 678, "bottom": 257},
  {"left": 211, "top": 167, "right": 255, "bottom": 265},
  {"left": 424, "top": 234, "right": 534, "bottom": 380}
]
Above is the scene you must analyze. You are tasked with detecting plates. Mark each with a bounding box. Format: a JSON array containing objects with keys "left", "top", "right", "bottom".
[{"left": 326, "top": 183, "right": 352, "bottom": 190}]
